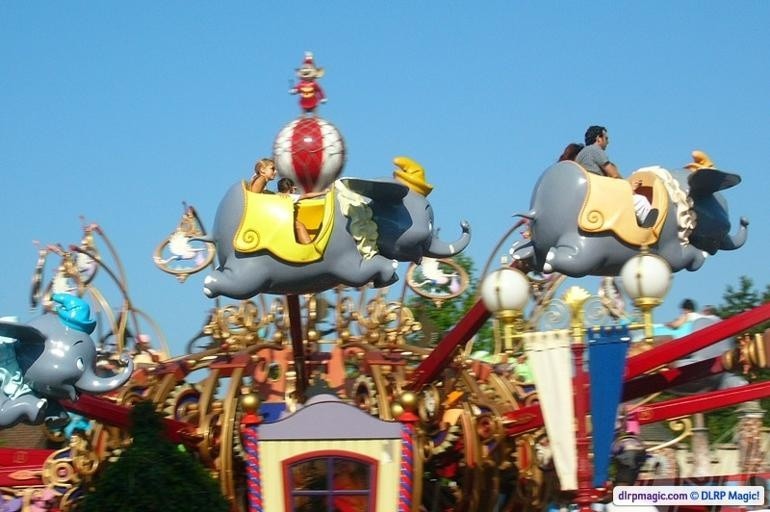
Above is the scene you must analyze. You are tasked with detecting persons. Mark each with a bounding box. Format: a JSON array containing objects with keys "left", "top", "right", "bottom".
[
  {"left": 558, "top": 143, "right": 585, "bottom": 161},
  {"left": 687, "top": 304, "right": 718, "bottom": 323},
  {"left": 664, "top": 299, "right": 699, "bottom": 329},
  {"left": 277, "top": 178, "right": 330, "bottom": 205},
  {"left": 249, "top": 158, "right": 312, "bottom": 244},
  {"left": 574, "top": 125, "right": 660, "bottom": 228}
]
